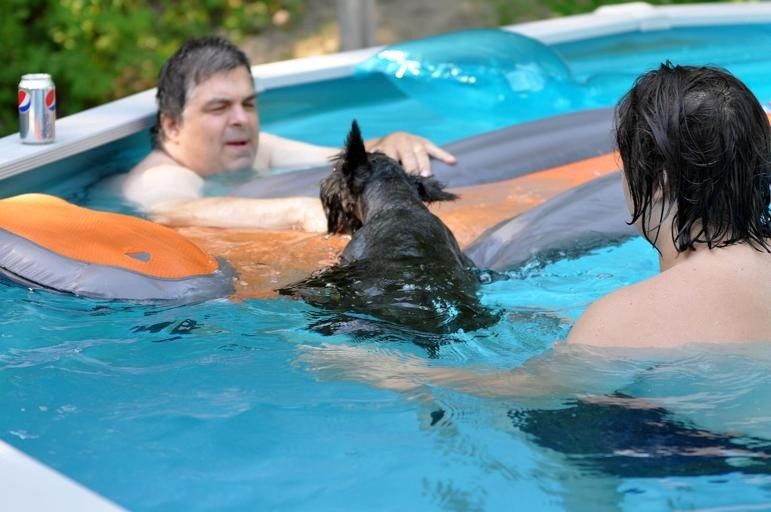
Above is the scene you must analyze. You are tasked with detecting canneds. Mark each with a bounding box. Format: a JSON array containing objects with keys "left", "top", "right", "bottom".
[{"left": 18, "top": 73, "right": 57, "bottom": 145}]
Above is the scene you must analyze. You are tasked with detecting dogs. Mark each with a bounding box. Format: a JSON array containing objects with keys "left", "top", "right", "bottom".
[{"left": 275, "top": 118, "right": 771, "bottom": 481}]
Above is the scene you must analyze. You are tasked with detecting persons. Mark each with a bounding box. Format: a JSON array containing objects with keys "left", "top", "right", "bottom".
[
  {"left": 121, "top": 36, "right": 457, "bottom": 234},
  {"left": 295, "top": 61, "right": 771, "bottom": 512}
]
